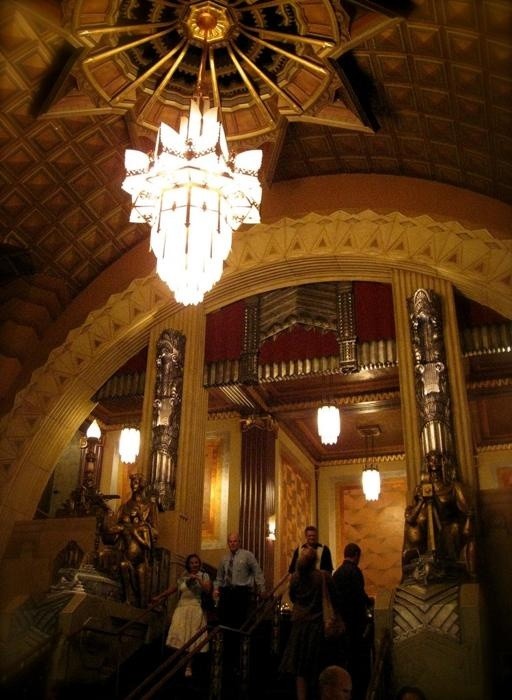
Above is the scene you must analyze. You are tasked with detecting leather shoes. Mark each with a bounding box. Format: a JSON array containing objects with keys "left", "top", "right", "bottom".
[{"left": 184, "top": 669, "right": 193, "bottom": 677}]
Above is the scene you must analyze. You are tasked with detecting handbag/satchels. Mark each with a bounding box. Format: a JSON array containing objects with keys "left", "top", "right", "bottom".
[
  {"left": 319, "top": 569, "right": 336, "bottom": 640},
  {"left": 200, "top": 590, "right": 216, "bottom": 610}
]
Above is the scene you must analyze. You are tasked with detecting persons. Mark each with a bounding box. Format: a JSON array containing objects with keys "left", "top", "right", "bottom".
[
  {"left": 211, "top": 531, "right": 266, "bottom": 627},
  {"left": 396, "top": 685, "right": 427, "bottom": 700},
  {"left": 328, "top": 542, "right": 375, "bottom": 666},
  {"left": 400, "top": 449, "right": 478, "bottom": 583},
  {"left": 98, "top": 471, "right": 160, "bottom": 604},
  {"left": 286, "top": 547, "right": 341, "bottom": 700},
  {"left": 150, "top": 554, "right": 213, "bottom": 678},
  {"left": 319, "top": 665, "right": 353, "bottom": 700},
  {"left": 287, "top": 526, "right": 333, "bottom": 575}
]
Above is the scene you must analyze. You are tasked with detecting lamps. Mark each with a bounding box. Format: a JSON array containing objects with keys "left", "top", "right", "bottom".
[
  {"left": 121, "top": 11, "right": 267, "bottom": 308},
  {"left": 357, "top": 423, "right": 383, "bottom": 503},
  {"left": 117, "top": 394, "right": 142, "bottom": 466},
  {"left": 82, "top": 417, "right": 102, "bottom": 490},
  {"left": 315, "top": 375, "right": 344, "bottom": 446}
]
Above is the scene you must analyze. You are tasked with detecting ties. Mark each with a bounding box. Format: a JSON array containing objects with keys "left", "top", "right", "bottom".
[{"left": 227, "top": 555, "right": 234, "bottom": 591}]
